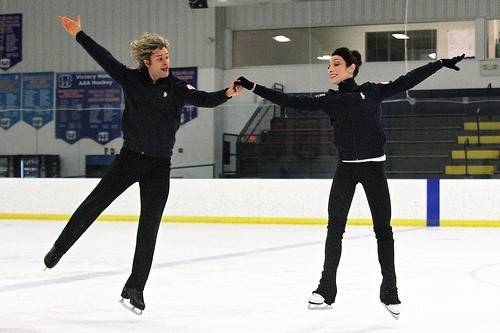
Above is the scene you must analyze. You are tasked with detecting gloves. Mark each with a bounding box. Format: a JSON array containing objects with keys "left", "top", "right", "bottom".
[
  {"left": 440, "top": 53, "right": 465, "bottom": 71},
  {"left": 233, "top": 76, "right": 254, "bottom": 93}
]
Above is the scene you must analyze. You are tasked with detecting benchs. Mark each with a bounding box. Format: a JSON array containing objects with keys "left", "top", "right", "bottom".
[{"left": 238, "top": 87, "right": 500, "bottom": 180}]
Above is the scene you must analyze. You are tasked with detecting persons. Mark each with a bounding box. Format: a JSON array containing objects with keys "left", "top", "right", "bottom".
[
  {"left": 42, "top": 14, "right": 244, "bottom": 316},
  {"left": 234, "top": 47, "right": 466, "bottom": 320}
]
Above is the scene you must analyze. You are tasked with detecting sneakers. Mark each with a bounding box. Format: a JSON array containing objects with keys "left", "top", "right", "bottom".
[
  {"left": 119, "top": 284, "right": 145, "bottom": 316},
  {"left": 308, "top": 293, "right": 333, "bottom": 309},
  {"left": 387, "top": 304, "right": 399, "bottom": 320},
  {"left": 44, "top": 245, "right": 64, "bottom": 271}
]
